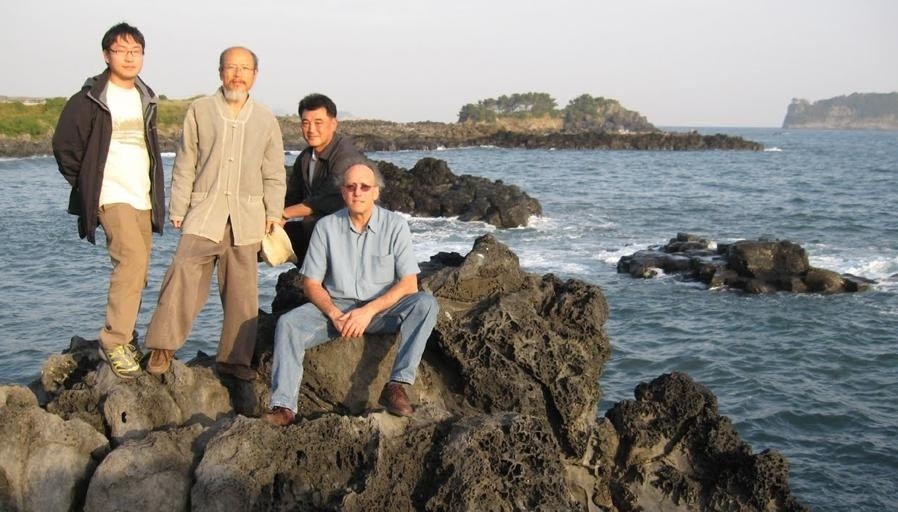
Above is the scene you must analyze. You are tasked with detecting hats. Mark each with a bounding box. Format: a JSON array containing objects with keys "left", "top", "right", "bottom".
[{"left": 262, "top": 222, "right": 298, "bottom": 267}]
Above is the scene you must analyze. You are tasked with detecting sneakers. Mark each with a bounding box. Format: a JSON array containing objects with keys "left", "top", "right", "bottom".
[
  {"left": 216, "top": 362, "right": 258, "bottom": 380},
  {"left": 147, "top": 348, "right": 174, "bottom": 376},
  {"left": 98, "top": 344, "right": 145, "bottom": 381}
]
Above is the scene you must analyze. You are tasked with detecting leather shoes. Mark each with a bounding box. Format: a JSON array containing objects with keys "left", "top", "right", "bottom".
[
  {"left": 261, "top": 407, "right": 294, "bottom": 425},
  {"left": 379, "top": 383, "right": 412, "bottom": 416}
]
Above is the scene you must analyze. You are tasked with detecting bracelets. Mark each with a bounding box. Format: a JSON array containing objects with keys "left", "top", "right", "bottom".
[{"left": 283, "top": 211, "right": 290, "bottom": 222}]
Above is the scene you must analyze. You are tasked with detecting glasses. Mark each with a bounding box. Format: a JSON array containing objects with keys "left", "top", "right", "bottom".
[
  {"left": 109, "top": 48, "right": 142, "bottom": 56},
  {"left": 345, "top": 182, "right": 376, "bottom": 192},
  {"left": 221, "top": 64, "right": 253, "bottom": 73}
]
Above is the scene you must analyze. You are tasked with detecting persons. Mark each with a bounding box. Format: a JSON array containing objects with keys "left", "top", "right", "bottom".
[
  {"left": 145, "top": 47, "right": 286, "bottom": 383},
  {"left": 260, "top": 157, "right": 440, "bottom": 425},
  {"left": 51, "top": 20, "right": 166, "bottom": 380},
  {"left": 278, "top": 93, "right": 366, "bottom": 268}
]
